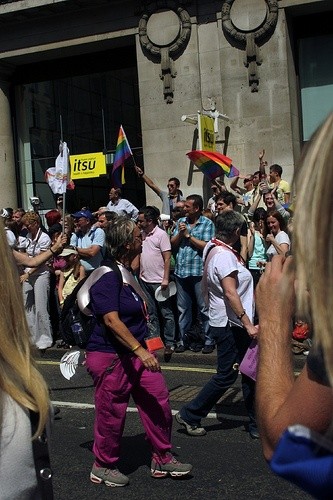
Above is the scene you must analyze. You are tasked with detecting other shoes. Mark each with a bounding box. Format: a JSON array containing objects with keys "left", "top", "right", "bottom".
[
  {"left": 203, "top": 344, "right": 215, "bottom": 353},
  {"left": 174, "top": 345, "right": 186, "bottom": 352},
  {"left": 192, "top": 343, "right": 204, "bottom": 351},
  {"left": 164, "top": 346, "right": 173, "bottom": 354},
  {"left": 57, "top": 341, "right": 69, "bottom": 348},
  {"left": 38, "top": 347, "right": 46, "bottom": 350}
]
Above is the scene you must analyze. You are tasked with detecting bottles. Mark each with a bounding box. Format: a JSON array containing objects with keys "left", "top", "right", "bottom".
[{"left": 69, "top": 309, "right": 87, "bottom": 346}]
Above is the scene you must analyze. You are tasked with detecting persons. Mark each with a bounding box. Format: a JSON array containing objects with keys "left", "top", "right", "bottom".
[
  {"left": 0, "top": 216, "right": 52, "bottom": 500},
  {"left": 256, "top": 108, "right": 333, "bottom": 460},
  {"left": 0, "top": 150, "right": 314, "bottom": 354},
  {"left": 84, "top": 220, "right": 192, "bottom": 487},
  {"left": 174, "top": 210, "right": 261, "bottom": 438}
]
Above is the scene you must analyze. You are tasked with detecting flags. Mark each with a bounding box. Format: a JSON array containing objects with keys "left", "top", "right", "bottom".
[
  {"left": 112, "top": 126, "right": 132, "bottom": 186},
  {"left": 45, "top": 142, "right": 70, "bottom": 193},
  {"left": 185, "top": 151, "right": 240, "bottom": 179}
]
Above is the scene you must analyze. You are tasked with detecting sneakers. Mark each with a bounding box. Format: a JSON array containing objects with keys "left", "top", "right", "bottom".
[
  {"left": 151, "top": 456, "right": 192, "bottom": 478},
  {"left": 90, "top": 461, "right": 128, "bottom": 487},
  {"left": 175, "top": 411, "right": 206, "bottom": 436},
  {"left": 251, "top": 430, "right": 261, "bottom": 438}
]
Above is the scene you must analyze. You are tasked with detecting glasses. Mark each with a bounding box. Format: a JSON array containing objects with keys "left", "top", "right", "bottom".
[
  {"left": 167, "top": 184, "right": 176, "bottom": 188},
  {"left": 135, "top": 234, "right": 142, "bottom": 240}
]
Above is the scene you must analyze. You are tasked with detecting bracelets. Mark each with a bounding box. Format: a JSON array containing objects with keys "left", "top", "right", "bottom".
[
  {"left": 140, "top": 173, "right": 143, "bottom": 177},
  {"left": 131, "top": 344, "right": 141, "bottom": 351},
  {"left": 237, "top": 311, "right": 245, "bottom": 319},
  {"left": 50, "top": 248, "right": 55, "bottom": 255},
  {"left": 28, "top": 272, "right": 31, "bottom": 276}
]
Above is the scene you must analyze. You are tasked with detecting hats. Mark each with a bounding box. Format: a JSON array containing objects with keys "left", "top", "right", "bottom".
[
  {"left": 70, "top": 210, "right": 95, "bottom": 224},
  {"left": 155, "top": 281, "right": 177, "bottom": 301},
  {"left": 57, "top": 248, "right": 78, "bottom": 257}
]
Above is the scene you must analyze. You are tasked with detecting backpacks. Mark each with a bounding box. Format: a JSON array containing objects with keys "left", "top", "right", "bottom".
[{"left": 60, "top": 261, "right": 123, "bottom": 349}]
